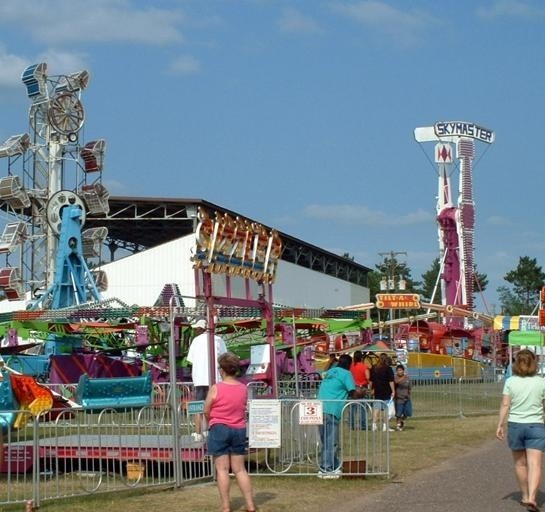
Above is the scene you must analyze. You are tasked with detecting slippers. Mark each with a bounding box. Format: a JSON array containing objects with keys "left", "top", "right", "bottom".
[{"left": 520, "top": 499, "right": 541, "bottom": 512}]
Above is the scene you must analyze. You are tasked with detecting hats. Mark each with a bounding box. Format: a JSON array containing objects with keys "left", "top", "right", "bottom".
[{"left": 191, "top": 319, "right": 207, "bottom": 329}]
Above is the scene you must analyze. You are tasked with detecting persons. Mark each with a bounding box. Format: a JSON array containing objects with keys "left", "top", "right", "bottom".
[
  {"left": 329, "top": 350, "right": 413, "bottom": 432},
  {"left": 204, "top": 352, "right": 257, "bottom": 512},
  {"left": 315, "top": 354, "right": 370, "bottom": 479},
  {"left": 453, "top": 342, "right": 462, "bottom": 355},
  {"left": 187, "top": 319, "right": 228, "bottom": 441},
  {"left": 496, "top": 349, "right": 545, "bottom": 512},
  {"left": 504, "top": 357, "right": 515, "bottom": 380}
]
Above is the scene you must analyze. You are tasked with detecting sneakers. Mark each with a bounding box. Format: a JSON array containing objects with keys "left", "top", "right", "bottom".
[
  {"left": 191, "top": 431, "right": 209, "bottom": 442},
  {"left": 317, "top": 470, "right": 343, "bottom": 479},
  {"left": 372, "top": 425, "right": 379, "bottom": 432},
  {"left": 382, "top": 426, "right": 395, "bottom": 432}
]
